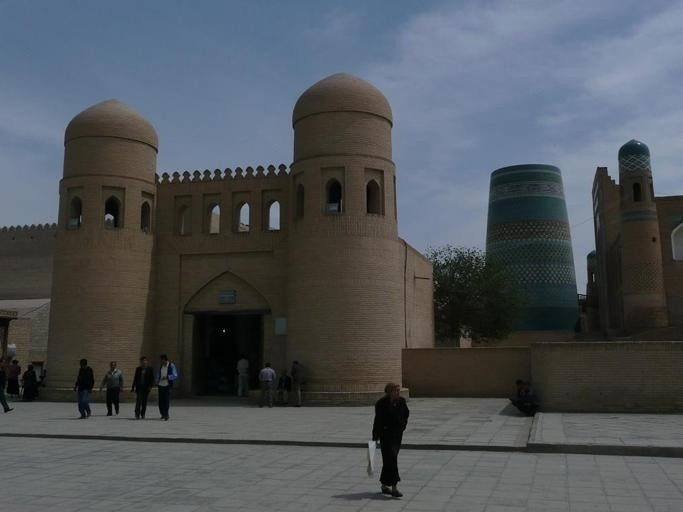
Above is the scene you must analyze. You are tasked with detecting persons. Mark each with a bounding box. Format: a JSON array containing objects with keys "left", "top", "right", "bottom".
[
  {"left": 5, "top": 359, "right": 21, "bottom": 399},
  {"left": 0, "top": 357, "right": 13, "bottom": 413},
  {"left": 129, "top": 357, "right": 154, "bottom": 419},
  {"left": 236, "top": 353, "right": 249, "bottom": 398},
  {"left": 370, "top": 382, "right": 409, "bottom": 497},
  {"left": 153, "top": 353, "right": 178, "bottom": 421},
  {"left": 257, "top": 362, "right": 274, "bottom": 409},
  {"left": 99, "top": 360, "right": 124, "bottom": 416},
  {"left": 19, "top": 364, "right": 37, "bottom": 402},
  {"left": 290, "top": 359, "right": 306, "bottom": 406},
  {"left": 275, "top": 367, "right": 291, "bottom": 406},
  {"left": 72, "top": 358, "right": 93, "bottom": 420}
]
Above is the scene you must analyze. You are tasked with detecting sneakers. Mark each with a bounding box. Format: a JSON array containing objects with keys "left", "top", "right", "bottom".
[
  {"left": 106, "top": 411, "right": 120, "bottom": 415},
  {"left": 381, "top": 485, "right": 403, "bottom": 497},
  {"left": 135, "top": 411, "right": 146, "bottom": 418},
  {"left": 160, "top": 414, "right": 170, "bottom": 420},
  {"left": 78, "top": 412, "right": 91, "bottom": 418}
]
